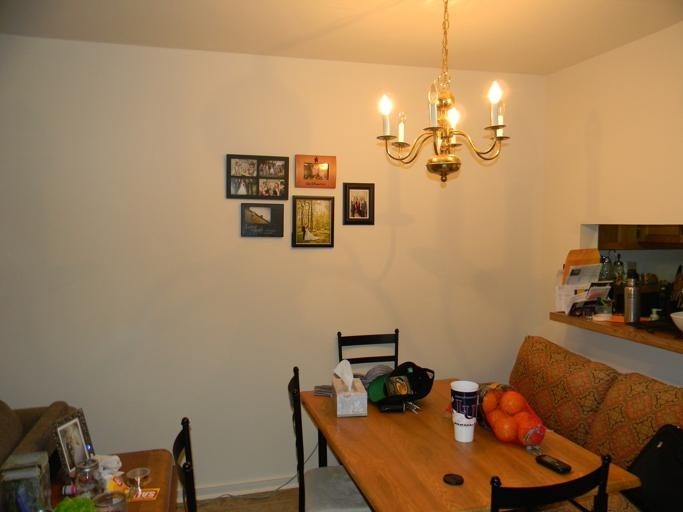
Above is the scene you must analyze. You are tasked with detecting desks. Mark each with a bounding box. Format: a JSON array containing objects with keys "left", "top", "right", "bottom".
[
  {"left": 298, "top": 377, "right": 644, "bottom": 510},
  {"left": 74, "top": 448, "right": 178, "bottom": 511}
]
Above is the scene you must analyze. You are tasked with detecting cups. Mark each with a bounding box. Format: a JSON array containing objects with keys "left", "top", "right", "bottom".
[
  {"left": 594, "top": 306, "right": 612, "bottom": 322},
  {"left": 582, "top": 310, "right": 592, "bottom": 321},
  {"left": 449, "top": 380, "right": 479, "bottom": 443},
  {"left": 94, "top": 491, "right": 127, "bottom": 511}
]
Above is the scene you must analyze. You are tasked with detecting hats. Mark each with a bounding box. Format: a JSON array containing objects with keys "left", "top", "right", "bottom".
[{"left": 367, "top": 361, "right": 434, "bottom": 406}]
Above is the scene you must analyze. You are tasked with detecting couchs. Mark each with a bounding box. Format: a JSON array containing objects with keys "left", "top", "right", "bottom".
[{"left": 0, "top": 398, "right": 73, "bottom": 511}]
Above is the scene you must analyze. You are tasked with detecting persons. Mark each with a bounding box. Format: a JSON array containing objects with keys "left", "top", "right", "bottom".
[
  {"left": 351, "top": 198, "right": 367, "bottom": 217},
  {"left": 232, "top": 160, "right": 286, "bottom": 196},
  {"left": 302, "top": 224, "right": 319, "bottom": 240}
]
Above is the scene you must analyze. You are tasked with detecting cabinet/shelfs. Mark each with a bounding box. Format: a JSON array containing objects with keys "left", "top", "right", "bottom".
[{"left": 596, "top": 225, "right": 682, "bottom": 252}]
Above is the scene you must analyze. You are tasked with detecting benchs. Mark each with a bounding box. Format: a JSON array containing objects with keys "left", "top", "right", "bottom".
[{"left": 505, "top": 333, "right": 682, "bottom": 512}]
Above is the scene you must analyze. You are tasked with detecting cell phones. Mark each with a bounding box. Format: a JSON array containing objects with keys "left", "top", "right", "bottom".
[{"left": 536, "top": 455, "right": 570, "bottom": 473}]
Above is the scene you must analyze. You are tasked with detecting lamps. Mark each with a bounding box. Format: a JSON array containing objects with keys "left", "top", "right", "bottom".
[{"left": 376, "top": 0, "right": 509, "bottom": 184}]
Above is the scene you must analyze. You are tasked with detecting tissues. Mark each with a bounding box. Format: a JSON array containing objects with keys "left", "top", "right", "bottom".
[{"left": 331, "top": 357, "right": 369, "bottom": 418}]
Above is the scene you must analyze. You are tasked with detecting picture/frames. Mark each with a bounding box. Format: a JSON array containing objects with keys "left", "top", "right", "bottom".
[
  {"left": 294, "top": 153, "right": 338, "bottom": 192},
  {"left": 49, "top": 407, "right": 98, "bottom": 479},
  {"left": 342, "top": 182, "right": 374, "bottom": 226},
  {"left": 292, "top": 195, "right": 336, "bottom": 248},
  {"left": 239, "top": 204, "right": 284, "bottom": 238},
  {"left": 224, "top": 150, "right": 289, "bottom": 203}
]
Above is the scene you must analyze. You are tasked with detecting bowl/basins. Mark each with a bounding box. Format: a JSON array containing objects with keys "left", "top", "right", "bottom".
[{"left": 669, "top": 311, "right": 682, "bottom": 332}]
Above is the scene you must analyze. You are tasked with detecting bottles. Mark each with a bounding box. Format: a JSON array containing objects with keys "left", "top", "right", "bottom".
[
  {"left": 623, "top": 278, "right": 640, "bottom": 324},
  {"left": 599, "top": 253, "right": 640, "bottom": 284},
  {"left": 74, "top": 460, "right": 102, "bottom": 497}
]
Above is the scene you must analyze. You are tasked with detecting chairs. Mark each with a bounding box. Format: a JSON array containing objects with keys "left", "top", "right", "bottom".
[
  {"left": 485, "top": 454, "right": 611, "bottom": 512},
  {"left": 286, "top": 366, "right": 372, "bottom": 512},
  {"left": 336, "top": 328, "right": 401, "bottom": 370},
  {"left": 171, "top": 416, "right": 202, "bottom": 512}
]
